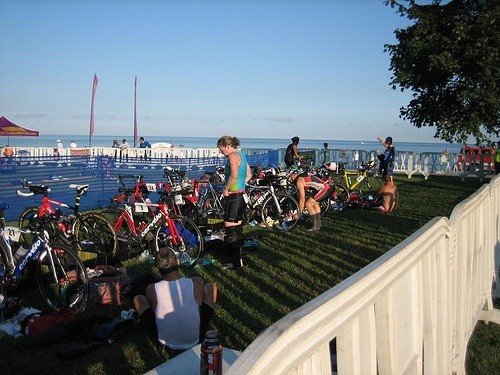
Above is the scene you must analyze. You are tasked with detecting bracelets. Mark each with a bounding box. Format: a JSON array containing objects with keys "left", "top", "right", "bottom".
[{"left": 224, "top": 185, "right": 230, "bottom": 191}]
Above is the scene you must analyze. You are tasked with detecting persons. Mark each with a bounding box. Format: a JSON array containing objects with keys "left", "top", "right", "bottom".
[
  {"left": 113, "top": 137, "right": 152, "bottom": 160},
  {"left": 56, "top": 139, "right": 64, "bottom": 148},
  {"left": 217, "top": 136, "right": 251, "bottom": 269},
  {"left": 70, "top": 140, "right": 77, "bottom": 148},
  {"left": 134, "top": 247, "right": 217, "bottom": 354},
  {"left": 284, "top": 136, "right": 400, "bottom": 232}
]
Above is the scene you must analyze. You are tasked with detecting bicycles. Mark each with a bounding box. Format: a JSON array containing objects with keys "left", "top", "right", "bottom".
[{"left": 0, "top": 160, "right": 398, "bottom": 317}]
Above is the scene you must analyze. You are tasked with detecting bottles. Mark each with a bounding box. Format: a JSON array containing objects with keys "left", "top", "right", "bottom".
[
  {"left": 200, "top": 331, "right": 223, "bottom": 375},
  {"left": 13, "top": 242, "right": 29, "bottom": 263}
]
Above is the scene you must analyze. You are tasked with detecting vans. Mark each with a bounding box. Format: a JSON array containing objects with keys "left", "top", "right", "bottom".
[{"left": 455, "top": 147, "right": 495, "bottom": 174}]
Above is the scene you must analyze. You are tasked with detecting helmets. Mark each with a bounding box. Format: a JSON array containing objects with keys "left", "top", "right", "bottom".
[
  {"left": 286, "top": 164, "right": 301, "bottom": 184},
  {"left": 292, "top": 137, "right": 299, "bottom": 142}
]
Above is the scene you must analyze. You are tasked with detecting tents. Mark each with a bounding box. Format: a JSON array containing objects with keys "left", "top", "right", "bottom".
[{"left": 0, "top": 116, "right": 39, "bottom": 148}]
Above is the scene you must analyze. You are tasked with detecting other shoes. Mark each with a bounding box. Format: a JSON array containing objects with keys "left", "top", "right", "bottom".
[{"left": 307, "top": 224, "right": 322, "bottom": 231}]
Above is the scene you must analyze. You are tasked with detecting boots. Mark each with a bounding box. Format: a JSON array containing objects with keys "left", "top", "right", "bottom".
[{"left": 220, "top": 225, "right": 244, "bottom": 270}]
